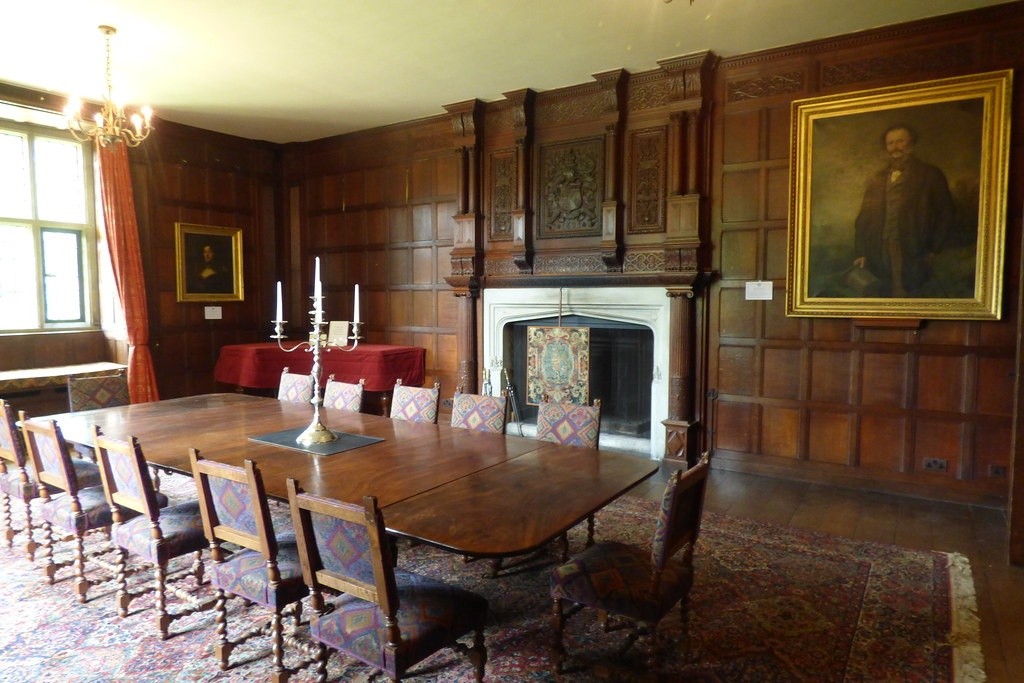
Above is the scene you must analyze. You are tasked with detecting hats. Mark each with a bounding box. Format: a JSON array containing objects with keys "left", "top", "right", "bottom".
[{"left": 837, "top": 265, "right": 876, "bottom": 295}]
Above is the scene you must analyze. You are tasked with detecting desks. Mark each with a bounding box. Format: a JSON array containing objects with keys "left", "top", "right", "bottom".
[
  {"left": 215, "top": 343, "right": 426, "bottom": 418},
  {"left": 15, "top": 392, "right": 661, "bottom": 683}
]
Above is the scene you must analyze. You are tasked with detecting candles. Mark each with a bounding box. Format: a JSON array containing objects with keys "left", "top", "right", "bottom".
[
  {"left": 276, "top": 282, "right": 284, "bottom": 322},
  {"left": 315, "top": 281, "right": 323, "bottom": 323},
  {"left": 353, "top": 282, "right": 362, "bottom": 323},
  {"left": 314, "top": 256, "right": 322, "bottom": 297}
]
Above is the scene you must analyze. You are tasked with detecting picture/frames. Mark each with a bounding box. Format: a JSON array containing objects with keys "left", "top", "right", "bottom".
[
  {"left": 784, "top": 69, "right": 1015, "bottom": 321},
  {"left": 175, "top": 223, "right": 245, "bottom": 302}
]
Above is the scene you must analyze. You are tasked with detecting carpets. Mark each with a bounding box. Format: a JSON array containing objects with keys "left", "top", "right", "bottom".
[{"left": 0, "top": 455, "right": 986, "bottom": 683}]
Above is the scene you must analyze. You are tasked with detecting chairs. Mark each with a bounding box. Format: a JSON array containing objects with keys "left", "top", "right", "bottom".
[
  {"left": 406, "top": 385, "right": 510, "bottom": 563},
  {"left": 67, "top": 369, "right": 173, "bottom": 491},
  {"left": 91, "top": 424, "right": 251, "bottom": 641},
  {"left": 548, "top": 450, "right": 713, "bottom": 683},
  {"left": 0, "top": 399, "right": 102, "bottom": 563},
  {"left": 390, "top": 378, "right": 443, "bottom": 420},
  {"left": 18, "top": 409, "right": 169, "bottom": 606},
  {"left": 489, "top": 394, "right": 602, "bottom": 580},
  {"left": 324, "top": 374, "right": 367, "bottom": 412},
  {"left": 276, "top": 366, "right": 314, "bottom": 401},
  {"left": 286, "top": 478, "right": 489, "bottom": 683},
  {"left": 187, "top": 447, "right": 311, "bottom": 683}
]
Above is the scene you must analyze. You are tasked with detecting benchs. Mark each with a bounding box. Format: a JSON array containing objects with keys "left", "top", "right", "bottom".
[{"left": 0, "top": 330, "right": 129, "bottom": 397}]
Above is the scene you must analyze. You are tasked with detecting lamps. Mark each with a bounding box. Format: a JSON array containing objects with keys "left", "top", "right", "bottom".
[{"left": 61, "top": 26, "right": 155, "bottom": 148}]
